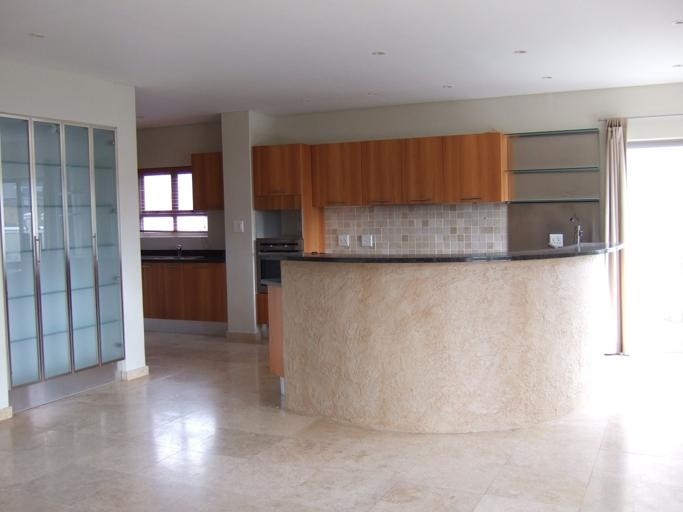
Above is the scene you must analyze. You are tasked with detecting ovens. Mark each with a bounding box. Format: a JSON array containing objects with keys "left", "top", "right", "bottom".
[{"left": 254, "top": 238, "right": 303, "bottom": 293}]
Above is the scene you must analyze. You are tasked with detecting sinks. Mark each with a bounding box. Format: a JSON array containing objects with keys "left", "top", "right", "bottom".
[
  {"left": 142, "top": 255, "right": 177, "bottom": 261},
  {"left": 172, "top": 255, "right": 205, "bottom": 260}
]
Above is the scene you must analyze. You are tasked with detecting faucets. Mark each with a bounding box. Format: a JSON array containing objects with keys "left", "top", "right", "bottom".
[
  {"left": 569, "top": 217, "right": 582, "bottom": 243},
  {"left": 176, "top": 243, "right": 183, "bottom": 257}
]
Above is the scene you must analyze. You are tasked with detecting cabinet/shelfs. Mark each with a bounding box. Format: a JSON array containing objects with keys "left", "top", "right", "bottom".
[
  {"left": 142, "top": 262, "right": 228, "bottom": 323},
  {"left": 310, "top": 133, "right": 509, "bottom": 207},
  {"left": 252, "top": 142, "right": 324, "bottom": 327},
  {"left": 190, "top": 153, "right": 224, "bottom": 212}
]
